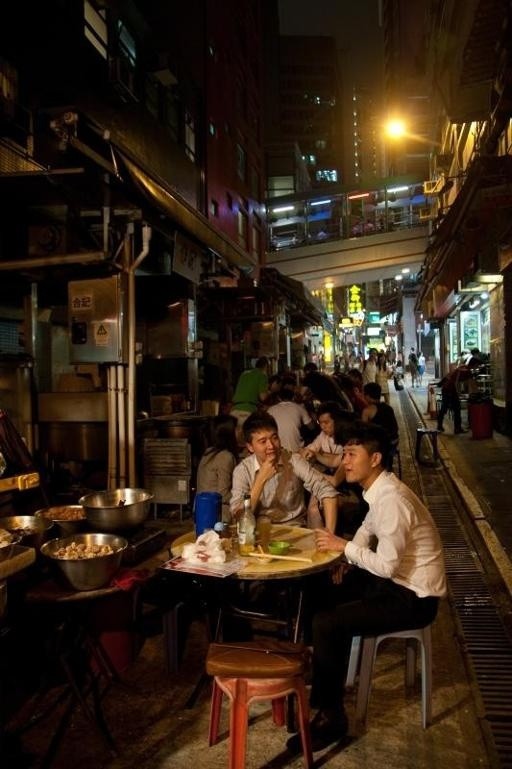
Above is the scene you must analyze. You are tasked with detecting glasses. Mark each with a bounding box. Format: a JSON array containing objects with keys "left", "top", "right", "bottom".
[{"left": 317, "top": 420, "right": 332, "bottom": 426}]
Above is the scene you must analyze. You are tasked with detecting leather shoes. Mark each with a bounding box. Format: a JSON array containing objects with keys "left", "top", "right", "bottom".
[{"left": 287, "top": 716, "right": 348, "bottom": 753}]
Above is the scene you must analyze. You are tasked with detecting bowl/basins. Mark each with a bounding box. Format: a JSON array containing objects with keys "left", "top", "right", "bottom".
[
  {"left": 41, "top": 533, "right": 128, "bottom": 591},
  {"left": 79, "top": 487, "right": 154, "bottom": 530},
  {"left": 33, "top": 504, "right": 87, "bottom": 537},
  {"left": 0, "top": 516, "right": 56, "bottom": 550},
  {"left": 266, "top": 540, "right": 290, "bottom": 556},
  {"left": 0, "top": 528, "right": 23, "bottom": 562}
]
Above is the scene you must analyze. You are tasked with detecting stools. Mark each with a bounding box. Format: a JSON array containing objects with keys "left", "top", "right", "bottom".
[
  {"left": 207, "top": 674, "right": 314, "bottom": 769},
  {"left": 345, "top": 623, "right": 433, "bottom": 735},
  {"left": 415, "top": 428, "right": 440, "bottom": 463},
  {"left": 389, "top": 449, "right": 402, "bottom": 480}
]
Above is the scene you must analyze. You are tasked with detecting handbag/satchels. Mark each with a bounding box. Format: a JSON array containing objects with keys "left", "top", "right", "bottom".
[{"left": 205, "top": 642, "right": 313, "bottom": 679}]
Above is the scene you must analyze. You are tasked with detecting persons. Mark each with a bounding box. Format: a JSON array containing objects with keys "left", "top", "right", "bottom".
[
  {"left": 437, "top": 347, "right": 489, "bottom": 435},
  {"left": 232, "top": 358, "right": 271, "bottom": 408},
  {"left": 266, "top": 347, "right": 399, "bottom": 445},
  {"left": 219, "top": 410, "right": 346, "bottom": 645},
  {"left": 197, "top": 414, "right": 242, "bottom": 504},
  {"left": 270, "top": 207, "right": 395, "bottom": 253},
  {"left": 408, "top": 347, "right": 425, "bottom": 388},
  {"left": 287, "top": 422, "right": 448, "bottom": 752}
]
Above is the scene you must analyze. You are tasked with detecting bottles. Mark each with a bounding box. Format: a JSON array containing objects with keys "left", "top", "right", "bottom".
[
  {"left": 195, "top": 492, "right": 222, "bottom": 539},
  {"left": 237, "top": 493, "right": 256, "bottom": 555},
  {"left": 219, "top": 522, "right": 233, "bottom": 554}
]
